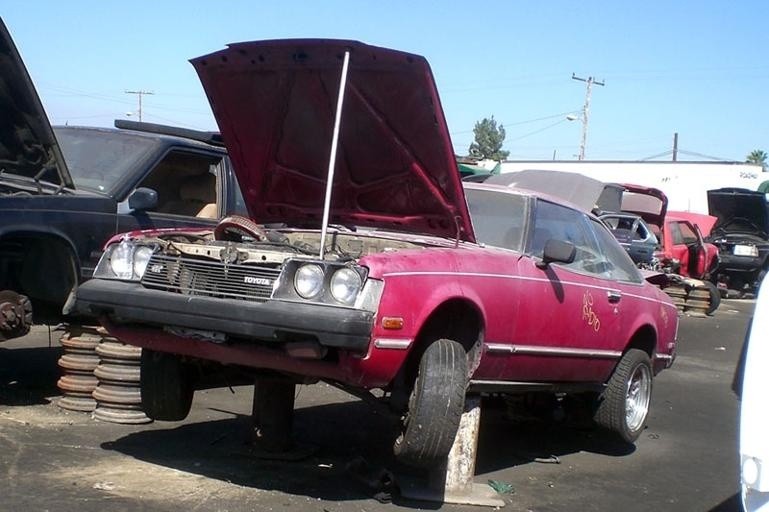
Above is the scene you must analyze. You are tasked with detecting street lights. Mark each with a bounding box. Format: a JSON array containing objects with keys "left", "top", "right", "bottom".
[
  {"left": 122, "top": 88, "right": 154, "bottom": 122},
  {"left": 564, "top": 69, "right": 605, "bottom": 162}
]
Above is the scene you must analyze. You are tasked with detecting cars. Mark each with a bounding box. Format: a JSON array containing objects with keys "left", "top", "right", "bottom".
[
  {"left": 66, "top": 35, "right": 681, "bottom": 464},
  {"left": 0, "top": 15, "right": 288, "bottom": 349}
]
